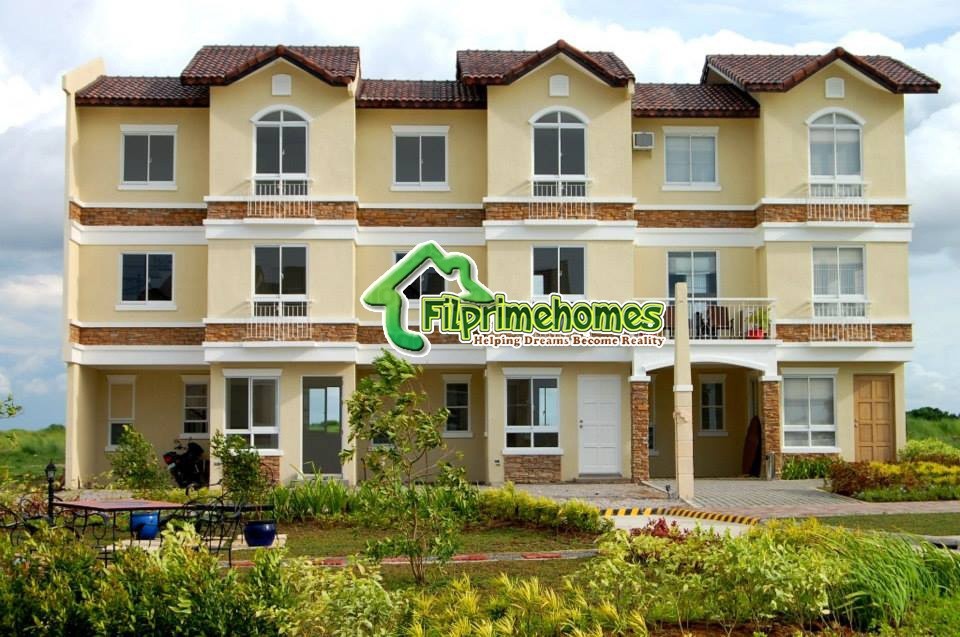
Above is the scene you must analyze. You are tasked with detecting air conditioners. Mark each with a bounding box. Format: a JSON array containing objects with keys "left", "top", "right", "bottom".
[{"left": 633, "top": 132, "right": 654, "bottom": 151}]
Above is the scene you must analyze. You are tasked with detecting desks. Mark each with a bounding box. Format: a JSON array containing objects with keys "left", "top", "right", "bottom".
[{"left": 53, "top": 500, "right": 195, "bottom": 568}]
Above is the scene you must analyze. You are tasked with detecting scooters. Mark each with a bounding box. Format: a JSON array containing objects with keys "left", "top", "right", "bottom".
[{"left": 164, "top": 436, "right": 207, "bottom": 488}]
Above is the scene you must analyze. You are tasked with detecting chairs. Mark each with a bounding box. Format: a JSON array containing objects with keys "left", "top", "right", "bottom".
[
  {"left": 661, "top": 304, "right": 674, "bottom": 336},
  {"left": 0, "top": 479, "right": 247, "bottom": 568},
  {"left": 706, "top": 306, "right": 734, "bottom": 336}
]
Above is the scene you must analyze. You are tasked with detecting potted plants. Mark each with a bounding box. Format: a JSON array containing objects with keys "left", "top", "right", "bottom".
[
  {"left": 103, "top": 423, "right": 173, "bottom": 540},
  {"left": 744, "top": 307, "right": 773, "bottom": 339},
  {"left": 210, "top": 428, "right": 278, "bottom": 547}
]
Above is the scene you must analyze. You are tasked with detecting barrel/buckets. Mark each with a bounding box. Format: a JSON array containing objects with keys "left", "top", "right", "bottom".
[
  {"left": 243, "top": 521, "right": 277, "bottom": 547},
  {"left": 131, "top": 512, "right": 158, "bottom": 540}
]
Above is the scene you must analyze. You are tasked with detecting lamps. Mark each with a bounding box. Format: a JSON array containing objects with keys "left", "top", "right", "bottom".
[{"left": 45, "top": 458, "right": 57, "bottom": 484}]
